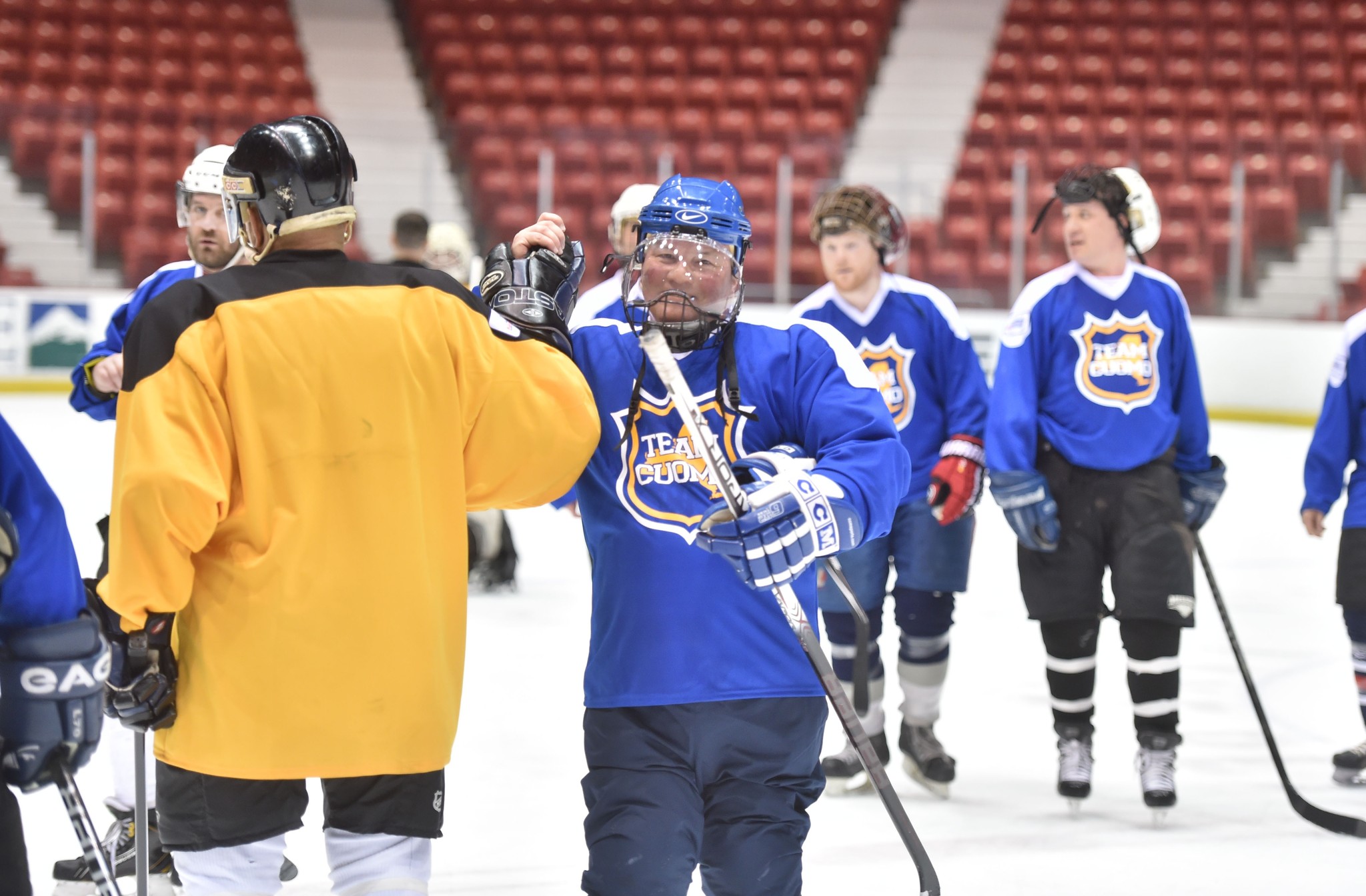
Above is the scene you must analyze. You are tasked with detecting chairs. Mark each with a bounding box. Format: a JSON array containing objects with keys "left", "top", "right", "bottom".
[{"left": 1, "top": 0, "right": 1365, "bottom": 322}]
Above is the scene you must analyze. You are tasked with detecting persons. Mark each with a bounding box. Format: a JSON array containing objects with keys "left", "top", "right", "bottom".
[
  {"left": 387, "top": 213, "right": 520, "bottom": 594},
  {"left": 0, "top": 412, "right": 113, "bottom": 896},
  {"left": 1299, "top": 307, "right": 1366, "bottom": 790},
  {"left": 788, "top": 186, "right": 991, "bottom": 800},
  {"left": 983, "top": 165, "right": 1226, "bottom": 823},
  {"left": 50, "top": 143, "right": 236, "bottom": 896},
  {"left": 568, "top": 183, "right": 660, "bottom": 330},
  {"left": 513, "top": 174, "right": 911, "bottom": 896},
  {"left": 96, "top": 114, "right": 602, "bottom": 896}
]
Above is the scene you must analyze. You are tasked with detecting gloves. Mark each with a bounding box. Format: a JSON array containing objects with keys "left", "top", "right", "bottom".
[
  {"left": 698, "top": 441, "right": 863, "bottom": 591},
  {"left": 92, "top": 602, "right": 178, "bottom": 729},
  {"left": 478, "top": 242, "right": 585, "bottom": 359},
  {"left": 1180, "top": 455, "right": 1225, "bottom": 532},
  {"left": 925, "top": 432, "right": 988, "bottom": 525},
  {"left": 1, "top": 614, "right": 110, "bottom": 790},
  {"left": 988, "top": 467, "right": 1060, "bottom": 554}
]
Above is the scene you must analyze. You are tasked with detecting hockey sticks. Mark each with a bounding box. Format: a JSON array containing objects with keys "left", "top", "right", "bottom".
[
  {"left": 1194, "top": 532, "right": 1366, "bottom": 839},
  {"left": 639, "top": 325, "right": 941, "bottom": 896},
  {"left": 51, "top": 761, "right": 123, "bottom": 896},
  {"left": 128, "top": 630, "right": 149, "bottom": 896},
  {"left": 825, "top": 558, "right": 872, "bottom": 718}
]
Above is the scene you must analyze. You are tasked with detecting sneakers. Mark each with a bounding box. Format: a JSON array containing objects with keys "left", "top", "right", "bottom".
[
  {"left": 1333, "top": 747, "right": 1366, "bottom": 787},
  {"left": 1057, "top": 733, "right": 1091, "bottom": 814},
  {"left": 1137, "top": 746, "right": 1178, "bottom": 823},
  {"left": 821, "top": 730, "right": 889, "bottom": 793},
  {"left": 898, "top": 716, "right": 955, "bottom": 799},
  {"left": 49, "top": 798, "right": 174, "bottom": 894}
]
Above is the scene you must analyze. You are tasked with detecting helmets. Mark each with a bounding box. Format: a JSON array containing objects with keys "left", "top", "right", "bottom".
[
  {"left": 609, "top": 183, "right": 663, "bottom": 228},
  {"left": 184, "top": 145, "right": 236, "bottom": 197},
  {"left": 221, "top": 115, "right": 356, "bottom": 238},
  {"left": 811, "top": 185, "right": 905, "bottom": 270},
  {"left": 622, "top": 176, "right": 751, "bottom": 352},
  {"left": 1055, "top": 165, "right": 1161, "bottom": 254}
]
